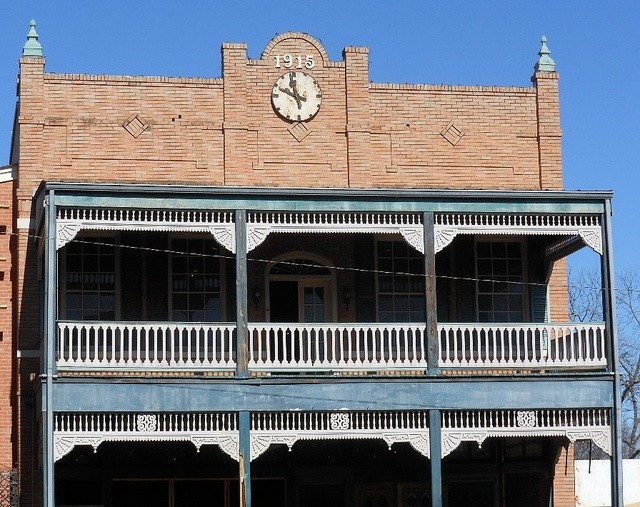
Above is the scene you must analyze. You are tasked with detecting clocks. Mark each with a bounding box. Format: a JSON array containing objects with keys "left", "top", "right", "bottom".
[{"left": 270, "top": 72, "right": 323, "bottom": 124}]
